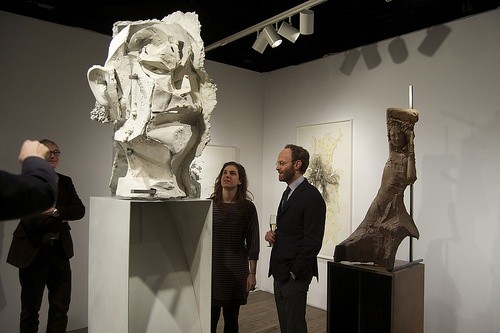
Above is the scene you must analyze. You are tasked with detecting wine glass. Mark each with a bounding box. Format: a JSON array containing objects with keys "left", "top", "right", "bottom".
[{"left": 267, "top": 214, "right": 276, "bottom": 247}]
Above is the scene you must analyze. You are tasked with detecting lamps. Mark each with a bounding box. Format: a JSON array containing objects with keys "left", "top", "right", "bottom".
[{"left": 252, "top": 7, "right": 314, "bottom": 55}]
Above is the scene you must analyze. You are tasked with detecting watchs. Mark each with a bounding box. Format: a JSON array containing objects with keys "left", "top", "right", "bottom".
[{"left": 52, "top": 209, "right": 59, "bottom": 217}]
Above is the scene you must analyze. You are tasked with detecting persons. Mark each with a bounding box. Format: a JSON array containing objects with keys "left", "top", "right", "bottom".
[
  {"left": 264, "top": 145, "right": 326, "bottom": 333},
  {"left": 0, "top": 139, "right": 59, "bottom": 221},
  {"left": 207, "top": 162, "right": 260, "bottom": 333},
  {"left": 5, "top": 138, "right": 87, "bottom": 333},
  {"left": 334, "top": 107, "right": 420, "bottom": 271}
]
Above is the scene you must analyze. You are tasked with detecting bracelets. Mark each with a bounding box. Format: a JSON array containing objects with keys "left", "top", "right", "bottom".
[{"left": 249, "top": 272, "right": 256, "bottom": 275}]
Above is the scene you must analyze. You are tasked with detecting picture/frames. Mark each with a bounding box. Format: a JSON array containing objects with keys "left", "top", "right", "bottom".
[{"left": 294, "top": 118, "right": 353, "bottom": 259}]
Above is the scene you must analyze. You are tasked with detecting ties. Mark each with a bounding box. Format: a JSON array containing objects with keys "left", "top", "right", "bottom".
[{"left": 279, "top": 185, "right": 291, "bottom": 209}]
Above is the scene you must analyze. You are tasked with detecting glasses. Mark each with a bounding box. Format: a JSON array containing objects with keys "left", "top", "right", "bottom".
[
  {"left": 276, "top": 159, "right": 293, "bottom": 166},
  {"left": 50, "top": 149, "right": 61, "bottom": 157}
]
